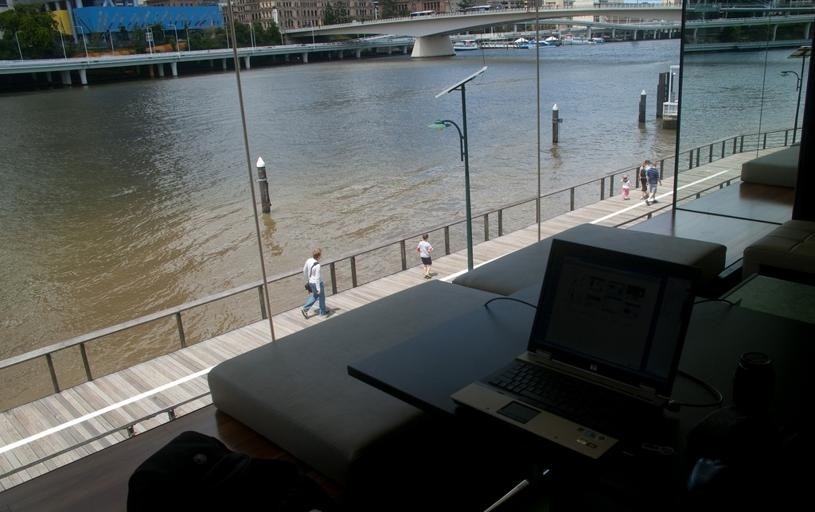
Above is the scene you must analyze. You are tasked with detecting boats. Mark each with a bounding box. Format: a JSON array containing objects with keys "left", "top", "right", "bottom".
[{"left": 453, "top": 40, "right": 480, "bottom": 50}]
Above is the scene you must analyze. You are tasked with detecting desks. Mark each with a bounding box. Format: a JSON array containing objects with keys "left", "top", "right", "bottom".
[
  {"left": 347, "top": 283, "right": 815, "bottom": 512},
  {"left": 719, "top": 273, "right": 815, "bottom": 326}
]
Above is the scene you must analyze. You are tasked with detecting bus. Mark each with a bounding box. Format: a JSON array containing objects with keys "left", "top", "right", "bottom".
[
  {"left": 410, "top": 10, "right": 434, "bottom": 17},
  {"left": 464, "top": 5, "right": 491, "bottom": 12}
]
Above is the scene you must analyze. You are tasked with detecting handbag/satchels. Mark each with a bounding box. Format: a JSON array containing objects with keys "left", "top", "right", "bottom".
[{"left": 305, "top": 283, "right": 318, "bottom": 292}]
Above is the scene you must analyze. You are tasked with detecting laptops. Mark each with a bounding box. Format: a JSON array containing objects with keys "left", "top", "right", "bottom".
[{"left": 450, "top": 237, "right": 701, "bottom": 460}]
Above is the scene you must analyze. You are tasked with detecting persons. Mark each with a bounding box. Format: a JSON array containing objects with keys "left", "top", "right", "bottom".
[
  {"left": 300, "top": 246, "right": 331, "bottom": 321},
  {"left": 645, "top": 159, "right": 661, "bottom": 206},
  {"left": 638, "top": 162, "right": 649, "bottom": 200},
  {"left": 416, "top": 233, "right": 434, "bottom": 278},
  {"left": 621, "top": 174, "right": 632, "bottom": 200}
]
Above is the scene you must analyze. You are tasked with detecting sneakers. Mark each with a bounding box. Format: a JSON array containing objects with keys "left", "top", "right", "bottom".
[
  {"left": 424, "top": 273, "right": 432, "bottom": 279},
  {"left": 302, "top": 309, "right": 308, "bottom": 319}
]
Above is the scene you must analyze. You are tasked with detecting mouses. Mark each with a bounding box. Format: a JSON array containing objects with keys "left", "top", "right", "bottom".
[{"left": 684, "top": 458, "right": 741, "bottom": 509}]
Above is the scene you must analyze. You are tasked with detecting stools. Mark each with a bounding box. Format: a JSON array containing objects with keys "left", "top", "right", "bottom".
[{"left": 742, "top": 219, "right": 815, "bottom": 288}]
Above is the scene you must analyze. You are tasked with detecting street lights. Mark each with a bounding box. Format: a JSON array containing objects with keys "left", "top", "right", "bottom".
[
  {"left": 15, "top": 20, "right": 314, "bottom": 65},
  {"left": 427, "top": 66, "right": 489, "bottom": 271},
  {"left": 778, "top": 46, "right": 812, "bottom": 146}
]
